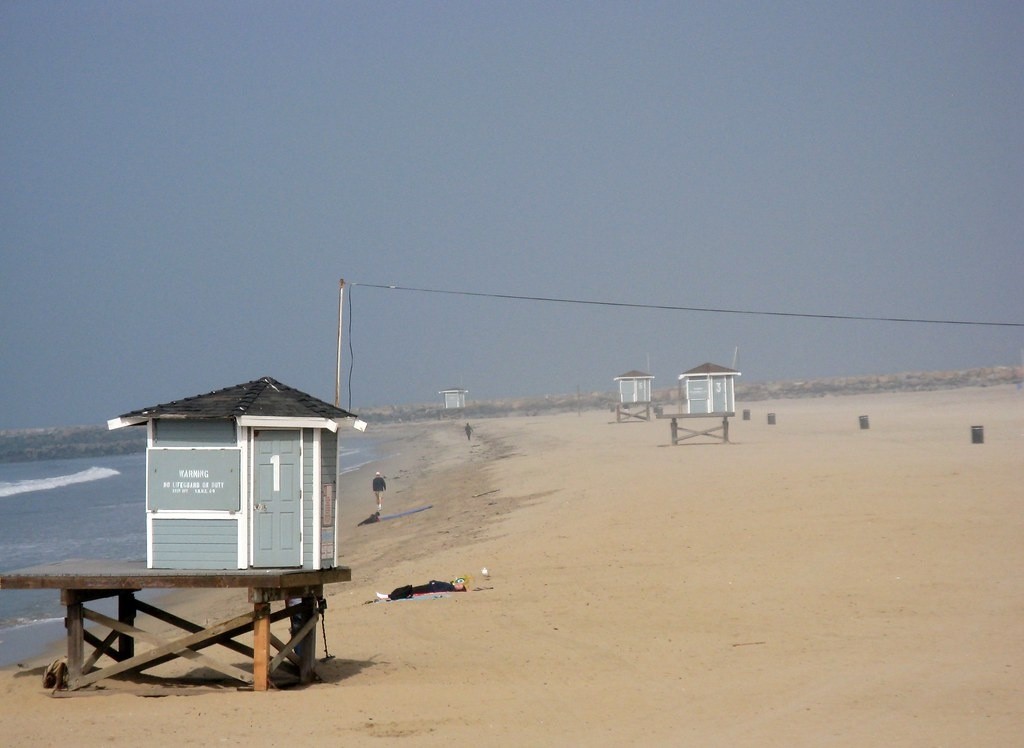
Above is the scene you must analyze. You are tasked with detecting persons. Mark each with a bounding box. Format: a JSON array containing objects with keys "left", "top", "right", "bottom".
[
  {"left": 373, "top": 472, "right": 386, "bottom": 511},
  {"left": 464, "top": 422, "right": 473, "bottom": 440},
  {"left": 358, "top": 512, "right": 380, "bottom": 527},
  {"left": 376, "top": 580, "right": 467, "bottom": 600}
]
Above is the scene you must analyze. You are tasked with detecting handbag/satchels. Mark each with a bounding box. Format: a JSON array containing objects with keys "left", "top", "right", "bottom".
[{"left": 388, "top": 585, "right": 412, "bottom": 600}]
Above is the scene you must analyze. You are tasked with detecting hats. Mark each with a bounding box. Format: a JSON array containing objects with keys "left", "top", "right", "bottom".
[{"left": 376, "top": 472, "right": 380, "bottom": 475}]
